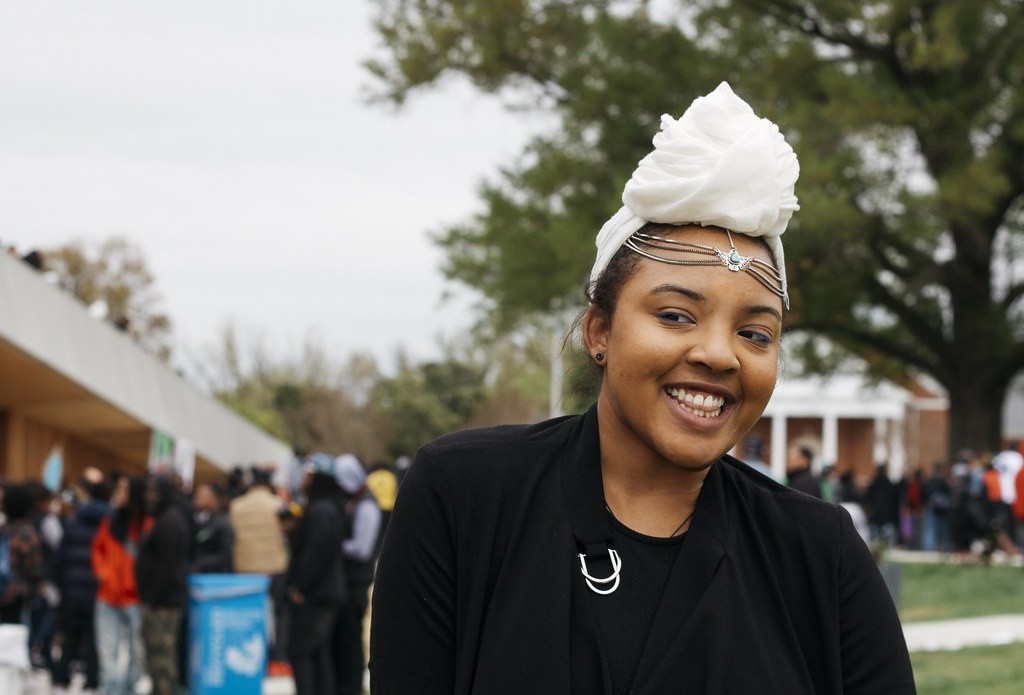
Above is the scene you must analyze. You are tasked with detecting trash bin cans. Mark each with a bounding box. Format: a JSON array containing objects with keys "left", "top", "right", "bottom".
[{"left": 179, "top": 572, "right": 273, "bottom": 695}]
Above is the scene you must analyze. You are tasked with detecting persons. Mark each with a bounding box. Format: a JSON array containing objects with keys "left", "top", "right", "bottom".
[
  {"left": 367, "top": 81, "right": 917, "bottom": 695},
  {"left": 742, "top": 439, "right": 1024, "bottom": 566},
  {"left": 0, "top": 454, "right": 409, "bottom": 695}
]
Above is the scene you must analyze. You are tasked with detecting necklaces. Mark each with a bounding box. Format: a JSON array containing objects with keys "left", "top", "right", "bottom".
[{"left": 604, "top": 500, "right": 695, "bottom": 538}]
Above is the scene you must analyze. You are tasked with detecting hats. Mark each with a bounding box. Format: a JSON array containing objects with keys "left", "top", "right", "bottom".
[{"left": 304, "top": 452, "right": 332, "bottom": 474}]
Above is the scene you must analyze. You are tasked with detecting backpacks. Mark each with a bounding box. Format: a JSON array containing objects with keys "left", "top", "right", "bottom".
[{"left": 9, "top": 511, "right": 49, "bottom": 585}]
